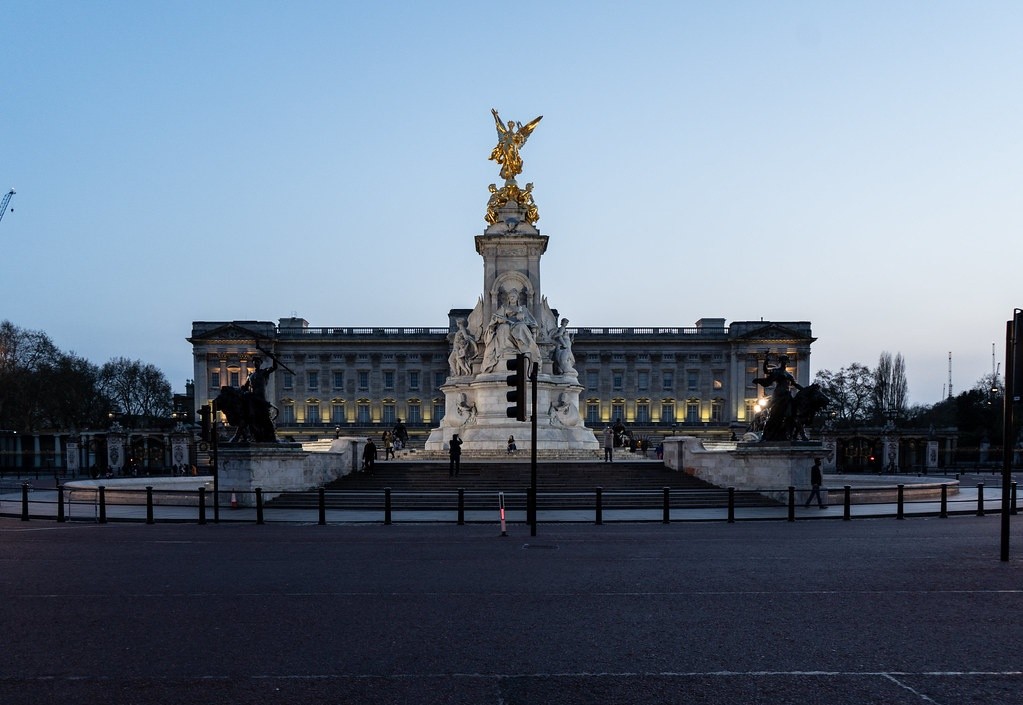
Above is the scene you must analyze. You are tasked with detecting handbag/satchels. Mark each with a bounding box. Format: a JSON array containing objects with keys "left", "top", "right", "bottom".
[{"left": 390, "top": 442, "right": 394, "bottom": 447}]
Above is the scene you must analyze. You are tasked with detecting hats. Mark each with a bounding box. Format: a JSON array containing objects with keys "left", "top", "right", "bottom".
[{"left": 452, "top": 434, "right": 458, "bottom": 438}]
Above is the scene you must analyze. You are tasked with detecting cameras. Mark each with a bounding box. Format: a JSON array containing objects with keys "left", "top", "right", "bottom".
[{"left": 607, "top": 426, "right": 610, "bottom": 428}]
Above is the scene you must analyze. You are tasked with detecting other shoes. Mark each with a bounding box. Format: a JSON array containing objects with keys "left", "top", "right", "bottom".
[
  {"left": 391, "top": 457, "right": 394, "bottom": 460},
  {"left": 805, "top": 505, "right": 810, "bottom": 508},
  {"left": 820, "top": 506, "right": 828, "bottom": 509},
  {"left": 448, "top": 474, "right": 453, "bottom": 478},
  {"left": 384, "top": 459, "right": 388, "bottom": 461},
  {"left": 454, "top": 474, "right": 457, "bottom": 477}
]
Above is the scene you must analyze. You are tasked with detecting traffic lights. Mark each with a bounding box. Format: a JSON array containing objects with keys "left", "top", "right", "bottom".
[
  {"left": 505, "top": 354, "right": 526, "bottom": 421},
  {"left": 197, "top": 404, "right": 212, "bottom": 443}
]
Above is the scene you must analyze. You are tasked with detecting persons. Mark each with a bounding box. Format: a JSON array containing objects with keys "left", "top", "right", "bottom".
[
  {"left": 229, "top": 353, "right": 279, "bottom": 444},
  {"left": 804, "top": 458, "right": 829, "bottom": 509},
  {"left": 483, "top": 121, "right": 539, "bottom": 228},
  {"left": 58, "top": 462, "right": 197, "bottom": 480},
  {"left": 753, "top": 355, "right": 806, "bottom": 442},
  {"left": 730, "top": 432, "right": 740, "bottom": 441},
  {"left": 506, "top": 434, "right": 517, "bottom": 456},
  {"left": 612, "top": 418, "right": 626, "bottom": 448},
  {"left": 447, "top": 287, "right": 581, "bottom": 377},
  {"left": 393, "top": 419, "right": 410, "bottom": 449},
  {"left": 361, "top": 430, "right": 402, "bottom": 472},
  {"left": 449, "top": 434, "right": 464, "bottom": 477},
  {"left": 602, "top": 424, "right": 663, "bottom": 460}
]
